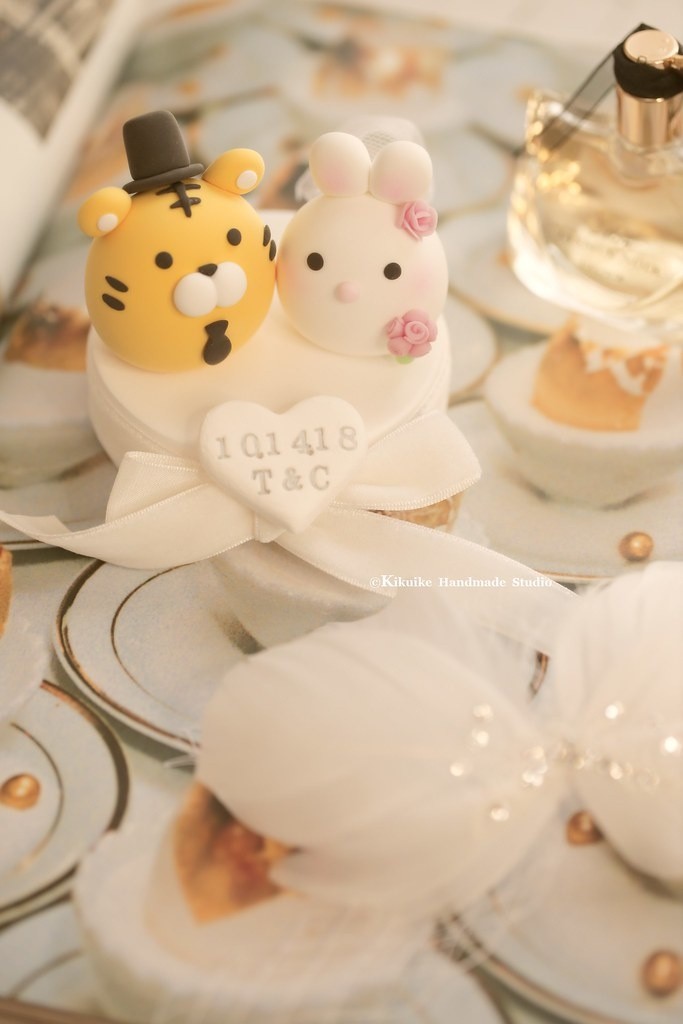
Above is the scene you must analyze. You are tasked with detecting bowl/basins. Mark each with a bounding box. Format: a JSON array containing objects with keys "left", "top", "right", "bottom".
[
  {"left": 564, "top": 564, "right": 683, "bottom": 884},
  {"left": 202, "top": 539, "right": 389, "bottom": 652},
  {"left": 481, "top": 343, "right": 683, "bottom": 506},
  {"left": 0, "top": 612, "right": 50, "bottom": 730}
]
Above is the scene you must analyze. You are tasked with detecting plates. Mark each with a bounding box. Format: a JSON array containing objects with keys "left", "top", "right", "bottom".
[
  {"left": 1, "top": 935, "right": 516, "bottom": 1024},
  {"left": 435, "top": 204, "right": 683, "bottom": 338},
  {"left": 0, "top": 680, "right": 131, "bottom": 921},
  {"left": 53, "top": 556, "right": 551, "bottom": 760},
  {"left": 444, "top": 810, "right": 683, "bottom": 1024}
]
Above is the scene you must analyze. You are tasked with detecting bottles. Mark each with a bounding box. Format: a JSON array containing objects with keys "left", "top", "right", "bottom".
[{"left": 506, "top": 32, "right": 683, "bottom": 317}]
